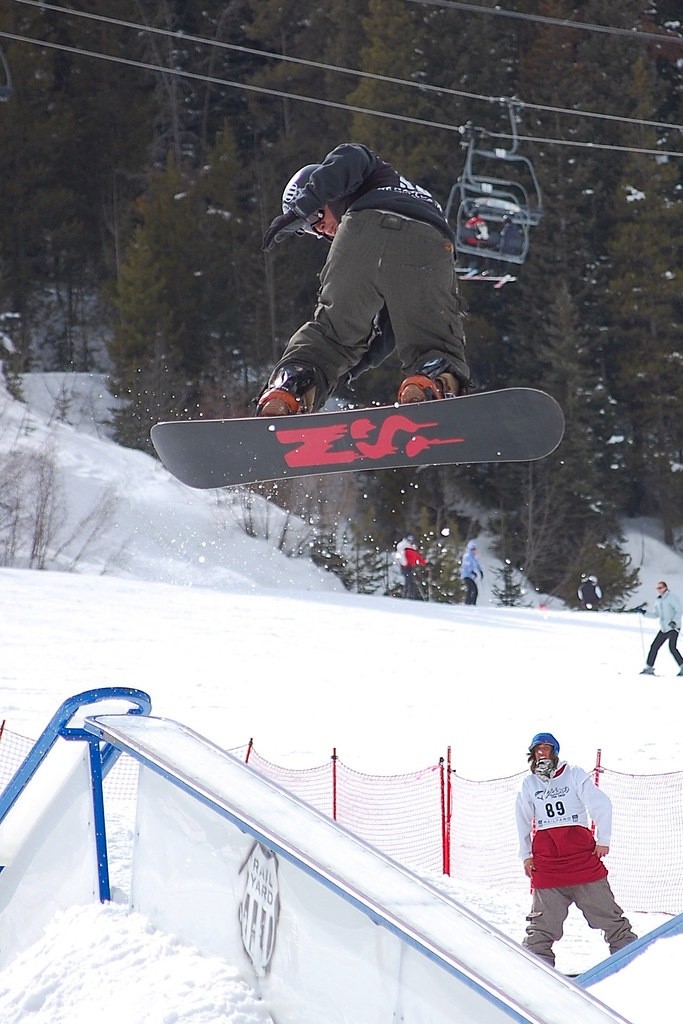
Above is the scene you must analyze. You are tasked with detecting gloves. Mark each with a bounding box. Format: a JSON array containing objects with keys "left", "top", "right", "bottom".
[
  {"left": 641, "top": 608, "right": 646, "bottom": 615},
  {"left": 328, "top": 374, "right": 349, "bottom": 397},
  {"left": 668, "top": 622, "right": 676, "bottom": 629},
  {"left": 261, "top": 208, "right": 299, "bottom": 254}
]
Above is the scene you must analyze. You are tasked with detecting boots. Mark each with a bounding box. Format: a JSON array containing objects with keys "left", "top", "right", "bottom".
[{"left": 254, "top": 363, "right": 317, "bottom": 418}]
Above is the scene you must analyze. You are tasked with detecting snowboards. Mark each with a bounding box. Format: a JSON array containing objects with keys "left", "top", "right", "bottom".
[{"left": 151, "top": 386, "right": 566, "bottom": 490}]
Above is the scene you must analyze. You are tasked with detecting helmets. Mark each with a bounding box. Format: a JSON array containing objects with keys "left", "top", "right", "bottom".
[
  {"left": 407, "top": 535, "right": 414, "bottom": 542},
  {"left": 589, "top": 575, "right": 598, "bottom": 582},
  {"left": 529, "top": 732, "right": 560, "bottom": 755},
  {"left": 468, "top": 541, "right": 477, "bottom": 550},
  {"left": 283, "top": 164, "right": 323, "bottom": 239}
]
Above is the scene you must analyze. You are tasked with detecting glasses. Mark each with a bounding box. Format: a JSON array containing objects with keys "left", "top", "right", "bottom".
[
  {"left": 657, "top": 587, "right": 664, "bottom": 589},
  {"left": 295, "top": 210, "right": 325, "bottom": 237}
]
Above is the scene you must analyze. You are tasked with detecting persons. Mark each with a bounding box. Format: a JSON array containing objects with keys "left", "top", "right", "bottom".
[
  {"left": 578, "top": 576, "right": 602, "bottom": 609},
  {"left": 490, "top": 216, "right": 521, "bottom": 276},
  {"left": 515, "top": 733, "right": 638, "bottom": 968},
  {"left": 398, "top": 535, "right": 426, "bottom": 599},
  {"left": 639, "top": 582, "right": 683, "bottom": 675},
  {"left": 465, "top": 210, "right": 489, "bottom": 270},
  {"left": 461, "top": 541, "right": 484, "bottom": 606},
  {"left": 258, "top": 142, "right": 471, "bottom": 415}
]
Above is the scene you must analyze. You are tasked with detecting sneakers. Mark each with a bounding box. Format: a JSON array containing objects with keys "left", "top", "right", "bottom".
[
  {"left": 639, "top": 668, "right": 656, "bottom": 675},
  {"left": 398, "top": 357, "right": 457, "bottom": 405},
  {"left": 677, "top": 667, "right": 683, "bottom": 676}
]
